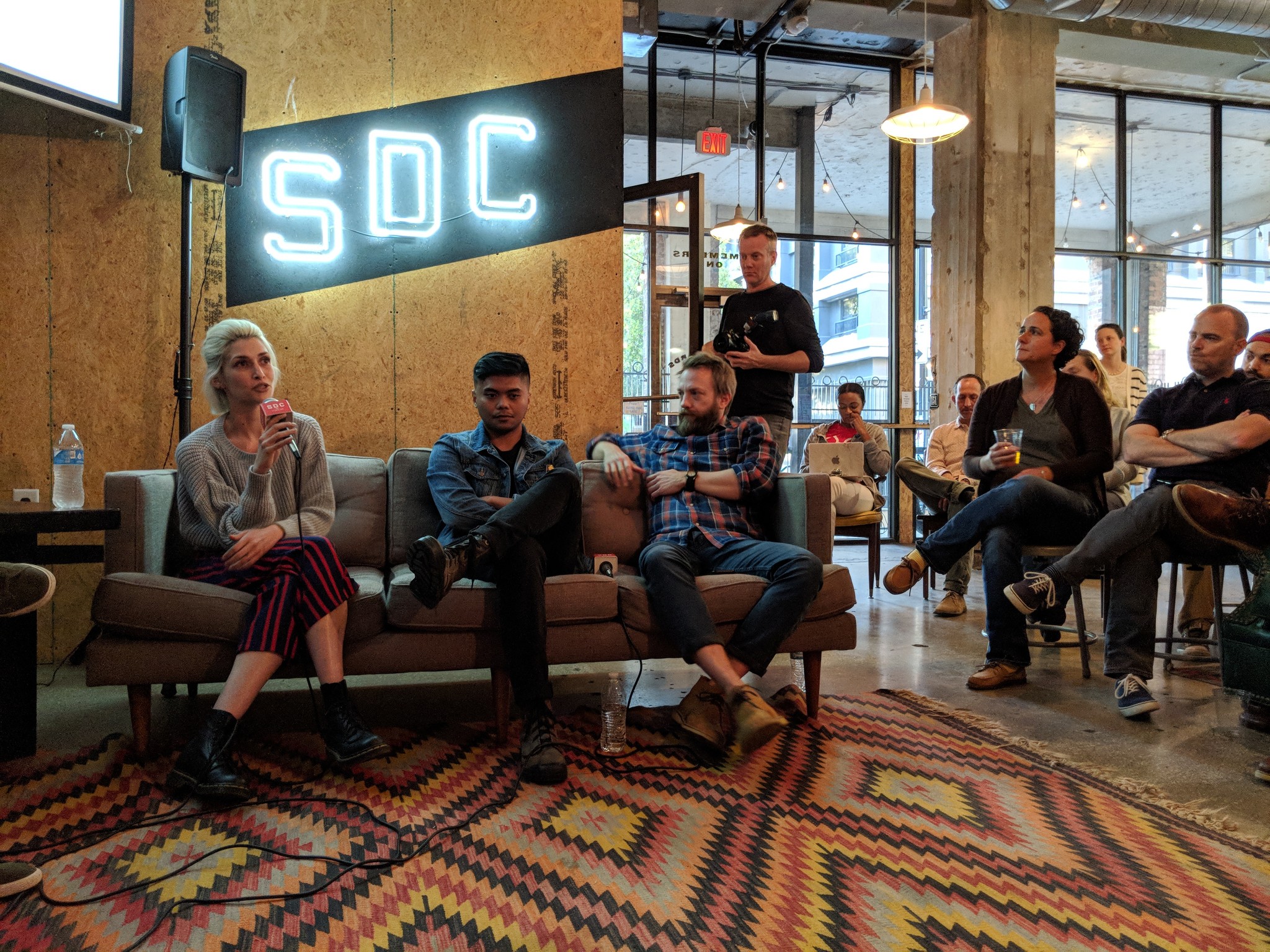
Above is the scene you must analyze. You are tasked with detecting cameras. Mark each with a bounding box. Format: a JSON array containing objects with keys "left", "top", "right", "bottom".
[{"left": 712, "top": 308, "right": 779, "bottom": 356}]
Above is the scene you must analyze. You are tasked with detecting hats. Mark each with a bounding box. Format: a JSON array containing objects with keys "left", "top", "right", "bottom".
[{"left": 1247, "top": 329, "right": 1270, "bottom": 346}]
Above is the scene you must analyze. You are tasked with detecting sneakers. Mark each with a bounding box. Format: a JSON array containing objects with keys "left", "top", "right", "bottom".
[
  {"left": 1003, "top": 570, "right": 1058, "bottom": 616},
  {"left": 933, "top": 590, "right": 967, "bottom": 615},
  {"left": 883, "top": 549, "right": 927, "bottom": 597},
  {"left": 966, "top": 658, "right": 1027, "bottom": 690},
  {"left": 1114, "top": 672, "right": 1158, "bottom": 717},
  {"left": 1176, "top": 627, "right": 1210, "bottom": 656}
]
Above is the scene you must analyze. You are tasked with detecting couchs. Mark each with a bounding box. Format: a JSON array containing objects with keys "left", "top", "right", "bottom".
[{"left": 86, "top": 448, "right": 858, "bottom": 760}]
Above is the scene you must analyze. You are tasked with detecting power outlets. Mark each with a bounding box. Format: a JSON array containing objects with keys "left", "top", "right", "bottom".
[{"left": 13, "top": 489, "right": 39, "bottom": 502}]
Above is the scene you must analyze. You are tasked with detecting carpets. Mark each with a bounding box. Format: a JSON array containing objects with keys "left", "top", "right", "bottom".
[{"left": 0, "top": 687, "right": 1270, "bottom": 951}]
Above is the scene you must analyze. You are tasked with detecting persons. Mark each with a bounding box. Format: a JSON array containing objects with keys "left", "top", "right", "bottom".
[
  {"left": 408, "top": 352, "right": 584, "bottom": 787},
  {"left": 895, "top": 374, "right": 986, "bottom": 616},
  {"left": 700, "top": 224, "right": 824, "bottom": 470},
  {"left": 883, "top": 306, "right": 1115, "bottom": 690},
  {"left": 799, "top": 383, "right": 892, "bottom": 556},
  {"left": 166, "top": 318, "right": 392, "bottom": 801},
  {"left": 587, "top": 351, "right": 823, "bottom": 754},
  {"left": 1003, "top": 305, "right": 1270, "bottom": 715},
  {"left": 1177, "top": 328, "right": 1269, "bottom": 653},
  {"left": 1094, "top": 324, "right": 1148, "bottom": 485},
  {"left": 1022, "top": 349, "right": 1140, "bottom": 640}
]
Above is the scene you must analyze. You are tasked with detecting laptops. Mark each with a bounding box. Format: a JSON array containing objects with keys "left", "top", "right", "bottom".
[{"left": 808, "top": 442, "right": 864, "bottom": 482}]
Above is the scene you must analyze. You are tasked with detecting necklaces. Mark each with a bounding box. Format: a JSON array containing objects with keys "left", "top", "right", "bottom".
[{"left": 1023, "top": 378, "right": 1050, "bottom": 411}]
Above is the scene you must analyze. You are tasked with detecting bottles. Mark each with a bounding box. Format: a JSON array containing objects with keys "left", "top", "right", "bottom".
[
  {"left": 600, "top": 672, "right": 627, "bottom": 753},
  {"left": 790, "top": 652, "right": 806, "bottom": 693},
  {"left": 52, "top": 424, "right": 85, "bottom": 508}
]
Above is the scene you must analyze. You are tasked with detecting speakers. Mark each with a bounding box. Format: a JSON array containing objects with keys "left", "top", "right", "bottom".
[{"left": 160, "top": 44, "right": 248, "bottom": 189}]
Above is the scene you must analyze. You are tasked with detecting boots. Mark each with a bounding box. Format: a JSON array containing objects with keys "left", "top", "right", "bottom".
[
  {"left": 518, "top": 699, "right": 566, "bottom": 783},
  {"left": 166, "top": 709, "right": 250, "bottom": 799},
  {"left": 408, "top": 535, "right": 492, "bottom": 608},
  {"left": 319, "top": 679, "right": 391, "bottom": 764}
]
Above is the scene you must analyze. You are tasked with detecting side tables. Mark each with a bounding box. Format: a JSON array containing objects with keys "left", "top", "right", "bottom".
[{"left": 0, "top": 500, "right": 121, "bottom": 760}]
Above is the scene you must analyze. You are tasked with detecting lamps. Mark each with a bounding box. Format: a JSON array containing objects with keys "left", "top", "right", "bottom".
[
  {"left": 881, "top": 1, "right": 969, "bottom": 144},
  {"left": 710, "top": 56, "right": 762, "bottom": 245},
  {"left": 737, "top": 126, "right": 769, "bottom": 151}
]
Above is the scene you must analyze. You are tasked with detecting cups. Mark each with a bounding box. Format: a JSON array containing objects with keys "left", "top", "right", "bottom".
[
  {"left": 993, "top": 428, "right": 1023, "bottom": 464},
  {"left": 1212, "top": 688, "right": 1250, "bottom": 738}
]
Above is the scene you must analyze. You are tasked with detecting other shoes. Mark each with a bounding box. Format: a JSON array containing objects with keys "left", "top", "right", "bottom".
[
  {"left": 726, "top": 687, "right": 787, "bottom": 753},
  {"left": 673, "top": 675, "right": 725, "bottom": 749},
  {"left": 1254, "top": 757, "right": 1270, "bottom": 783}
]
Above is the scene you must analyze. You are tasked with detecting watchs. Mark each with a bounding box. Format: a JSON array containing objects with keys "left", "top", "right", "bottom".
[
  {"left": 1039, "top": 467, "right": 1046, "bottom": 480},
  {"left": 1162, "top": 428, "right": 1174, "bottom": 441},
  {"left": 685, "top": 469, "right": 698, "bottom": 492}
]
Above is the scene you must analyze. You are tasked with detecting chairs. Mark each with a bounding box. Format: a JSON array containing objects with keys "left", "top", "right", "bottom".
[
  {"left": 835, "top": 474, "right": 887, "bottom": 599},
  {"left": 1153, "top": 554, "right": 1252, "bottom": 677},
  {"left": 981, "top": 545, "right": 1098, "bottom": 678},
  {"left": 916, "top": 514, "right": 981, "bottom": 600}
]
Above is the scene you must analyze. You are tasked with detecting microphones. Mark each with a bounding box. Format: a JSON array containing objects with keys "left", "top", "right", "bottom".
[
  {"left": 593, "top": 554, "right": 618, "bottom": 577},
  {"left": 263, "top": 397, "right": 302, "bottom": 460}
]
